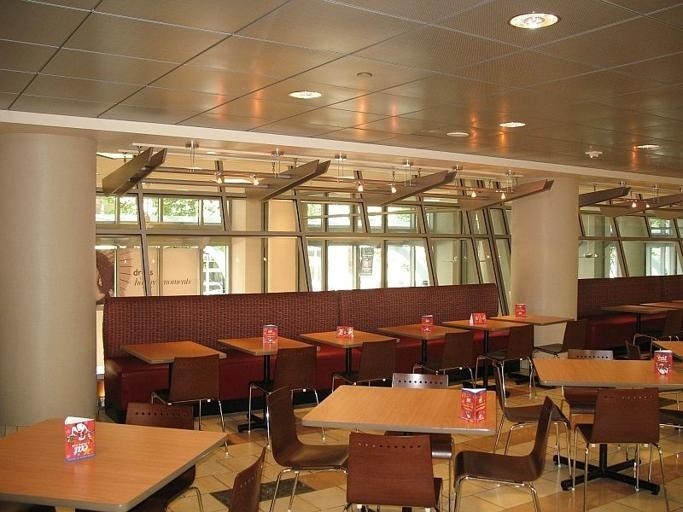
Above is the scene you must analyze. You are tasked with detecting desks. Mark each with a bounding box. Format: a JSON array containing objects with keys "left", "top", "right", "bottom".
[{"left": 0, "top": 416, "right": 227, "bottom": 512}]
